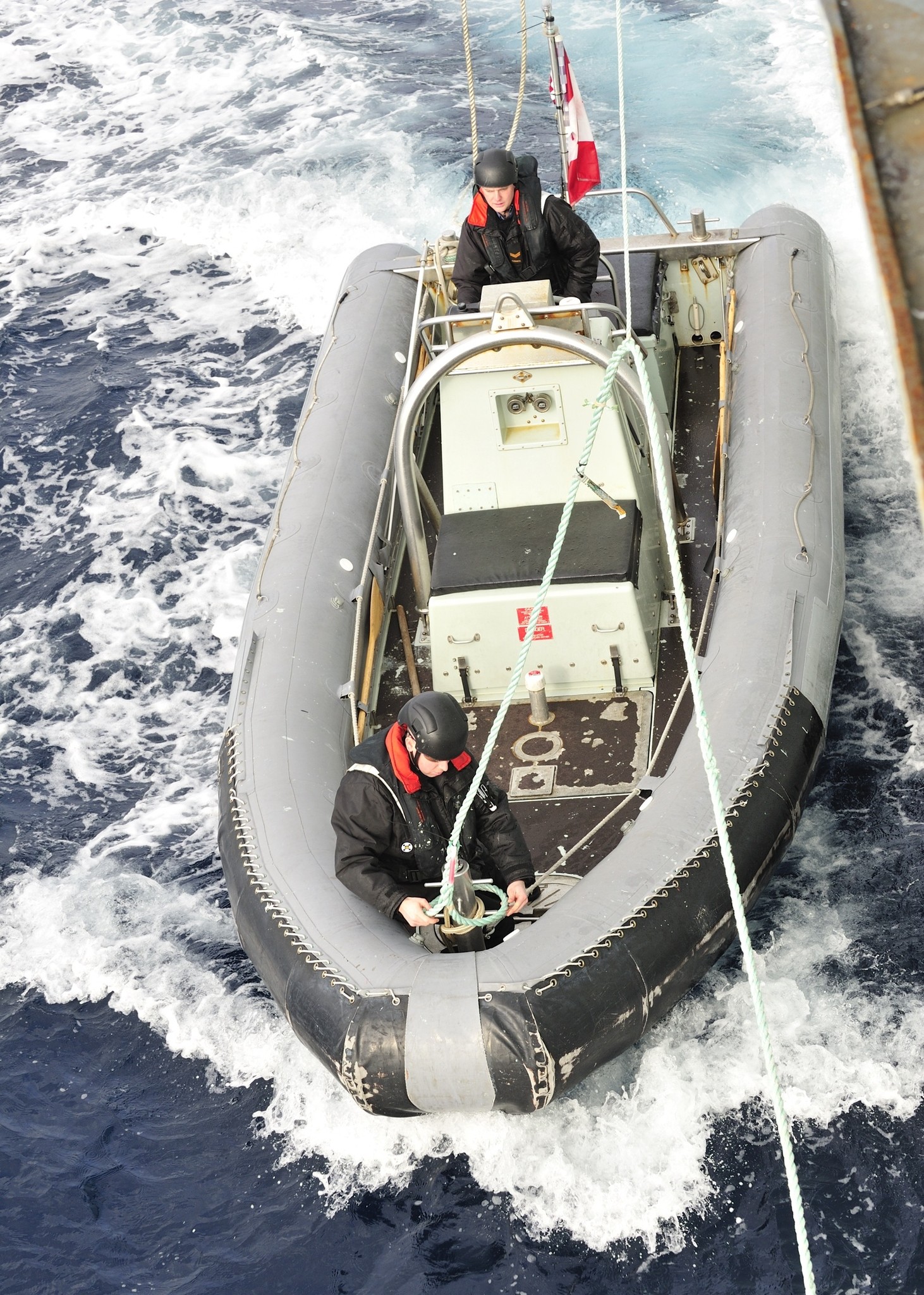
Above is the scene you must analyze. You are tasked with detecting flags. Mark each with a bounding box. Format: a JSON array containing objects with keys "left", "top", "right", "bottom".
[{"left": 549, "top": 40, "right": 600, "bottom": 206}]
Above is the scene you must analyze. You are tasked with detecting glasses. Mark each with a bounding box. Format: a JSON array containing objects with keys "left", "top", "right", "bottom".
[{"left": 424, "top": 754, "right": 436, "bottom": 761}]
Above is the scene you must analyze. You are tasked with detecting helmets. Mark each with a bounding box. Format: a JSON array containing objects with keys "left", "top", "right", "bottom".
[
  {"left": 397, "top": 691, "right": 468, "bottom": 760},
  {"left": 475, "top": 148, "right": 518, "bottom": 187}
]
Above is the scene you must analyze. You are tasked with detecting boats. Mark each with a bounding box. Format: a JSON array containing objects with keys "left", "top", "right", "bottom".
[{"left": 217, "top": 184, "right": 838, "bottom": 1116}]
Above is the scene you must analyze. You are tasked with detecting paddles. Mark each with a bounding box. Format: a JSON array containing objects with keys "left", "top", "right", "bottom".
[
  {"left": 355, "top": 576, "right": 384, "bottom": 741},
  {"left": 712, "top": 290, "right": 737, "bottom": 502}
]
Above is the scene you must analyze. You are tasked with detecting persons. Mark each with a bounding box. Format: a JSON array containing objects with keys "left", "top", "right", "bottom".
[
  {"left": 451, "top": 150, "right": 600, "bottom": 313},
  {"left": 331, "top": 691, "right": 541, "bottom": 954}
]
